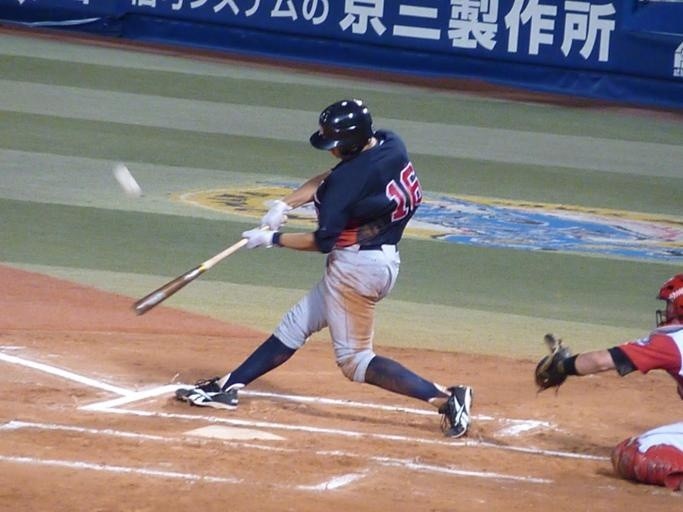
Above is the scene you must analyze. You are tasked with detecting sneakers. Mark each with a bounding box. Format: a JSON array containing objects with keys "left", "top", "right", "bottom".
[
  {"left": 172, "top": 376, "right": 247, "bottom": 410},
  {"left": 437, "top": 385, "right": 475, "bottom": 438}
]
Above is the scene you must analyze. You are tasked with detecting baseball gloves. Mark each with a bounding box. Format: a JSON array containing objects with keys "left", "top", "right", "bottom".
[{"left": 536, "top": 335, "right": 570, "bottom": 388}]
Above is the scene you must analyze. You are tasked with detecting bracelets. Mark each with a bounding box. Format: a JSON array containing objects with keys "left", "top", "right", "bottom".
[{"left": 272, "top": 233, "right": 282, "bottom": 248}]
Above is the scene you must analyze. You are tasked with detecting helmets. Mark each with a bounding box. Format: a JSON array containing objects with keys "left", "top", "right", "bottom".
[
  {"left": 309, "top": 97, "right": 375, "bottom": 153},
  {"left": 658, "top": 273, "right": 683, "bottom": 320}
]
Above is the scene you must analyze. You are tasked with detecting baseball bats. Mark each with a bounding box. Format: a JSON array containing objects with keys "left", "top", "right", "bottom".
[{"left": 132, "top": 215, "right": 288, "bottom": 316}]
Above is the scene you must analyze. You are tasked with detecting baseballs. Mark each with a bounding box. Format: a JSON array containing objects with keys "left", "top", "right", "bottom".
[{"left": 114, "top": 168, "right": 137, "bottom": 193}]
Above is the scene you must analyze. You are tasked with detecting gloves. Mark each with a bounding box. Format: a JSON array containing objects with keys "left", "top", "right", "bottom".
[
  {"left": 258, "top": 199, "right": 294, "bottom": 230},
  {"left": 241, "top": 227, "right": 278, "bottom": 252}
]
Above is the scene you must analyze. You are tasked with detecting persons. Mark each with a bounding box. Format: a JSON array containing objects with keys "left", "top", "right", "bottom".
[
  {"left": 535, "top": 271, "right": 683, "bottom": 492},
  {"left": 172, "top": 98, "right": 475, "bottom": 440}
]
